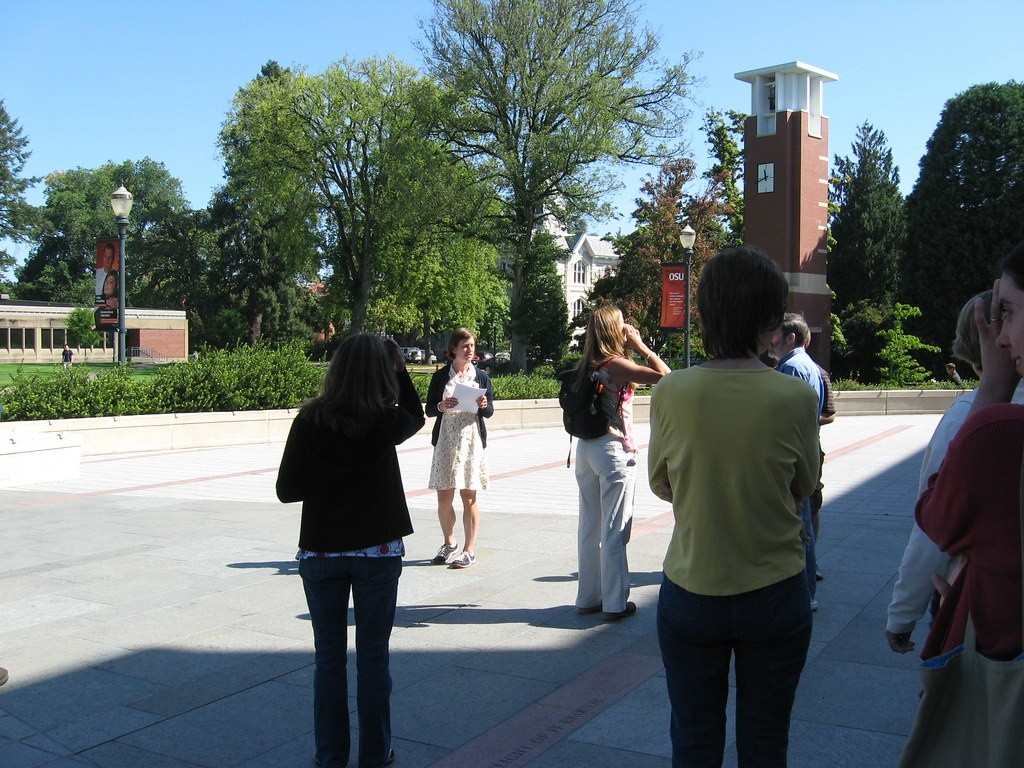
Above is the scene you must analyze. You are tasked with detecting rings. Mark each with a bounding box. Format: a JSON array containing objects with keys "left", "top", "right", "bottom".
[{"left": 991, "top": 317, "right": 1002, "bottom": 321}]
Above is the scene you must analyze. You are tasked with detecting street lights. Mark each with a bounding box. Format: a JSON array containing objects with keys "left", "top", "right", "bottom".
[
  {"left": 493, "top": 319, "right": 497, "bottom": 371},
  {"left": 678, "top": 224, "right": 693, "bottom": 369},
  {"left": 109, "top": 181, "right": 132, "bottom": 371}
]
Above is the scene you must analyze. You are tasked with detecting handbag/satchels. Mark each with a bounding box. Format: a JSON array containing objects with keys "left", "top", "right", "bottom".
[{"left": 894, "top": 646, "right": 1024, "bottom": 768}]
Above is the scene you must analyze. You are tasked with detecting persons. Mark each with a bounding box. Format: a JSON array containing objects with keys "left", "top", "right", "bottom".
[
  {"left": 886, "top": 290, "right": 1024, "bottom": 653},
  {"left": 62, "top": 344, "right": 73, "bottom": 371},
  {"left": 945, "top": 363, "right": 961, "bottom": 385},
  {"left": 575, "top": 308, "right": 671, "bottom": 620},
  {"left": 192, "top": 350, "right": 198, "bottom": 360},
  {"left": 805, "top": 329, "right": 835, "bottom": 579},
  {"left": 916, "top": 243, "right": 1024, "bottom": 699},
  {"left": 96, "top": 244, "right": 120, "bottom": 328},
  {"left": 649, "top": 248, "right": 819, "bottom": 768},
  {"left": 769, "top": 313, "right": 825, "bottom": 609},
  {"left": 425, "top": 328, "right": 494, "bottom": 567},
  {"left": 277, "top": 335, "right": 426, "bottom": 768}
]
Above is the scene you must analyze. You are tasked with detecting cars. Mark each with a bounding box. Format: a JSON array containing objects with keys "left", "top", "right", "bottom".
[
  {"left": 471, "top": 351, "right": 510, "bottom": 369},
  {"left": 420, "top": 349, "right": 437, "bottom": 364}
]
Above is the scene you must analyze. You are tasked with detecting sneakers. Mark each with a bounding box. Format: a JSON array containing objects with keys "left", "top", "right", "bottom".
[
  {"left": 433, "top": 544, "right": 458, "bottom": 564},
  {"left": 452, "top": 551, "right": 475, "bottom": 568}
]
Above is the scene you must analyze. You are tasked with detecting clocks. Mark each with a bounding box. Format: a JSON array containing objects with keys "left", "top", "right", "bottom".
[{"left": 757, "top": 163, "right": 775, "bottom": 194}]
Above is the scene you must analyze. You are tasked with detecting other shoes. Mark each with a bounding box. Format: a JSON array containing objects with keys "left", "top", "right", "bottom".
[
  {"left": 815, "top": 565, "right": 824, "bottom": 579},
  {"left": 314, "top": 751, "right": 321, "bottom": 764},
  {"left": 810, "top": 597, "right": 818, "bottom": 610},
  {"left": 0, "top": 667, "right": 9, "bottom": 686},
  {"left": 579, "top": 601, "right": 603, "bottom": 615},
  {"left": 385, "top": 746, "right": 395, "bottom": 764},
  {"left": 603, "top": 602, "right": 636, "bottom": 619}
]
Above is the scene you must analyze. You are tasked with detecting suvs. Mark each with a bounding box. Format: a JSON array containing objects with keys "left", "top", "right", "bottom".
[{"left": 400, "top": 347, "right": 422, "bottom": 364}]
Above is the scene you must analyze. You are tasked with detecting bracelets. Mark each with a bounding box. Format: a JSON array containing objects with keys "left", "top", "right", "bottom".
[
  {"left": 438, "top": 402, "right": 447, "bottom": 412},
  {"left": 646, "top": 352, "right": 656, "bottom": 360}
]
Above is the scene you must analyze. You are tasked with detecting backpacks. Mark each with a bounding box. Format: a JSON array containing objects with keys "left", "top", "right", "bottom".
[{"left": 558, "top": 359, "right": 627, "bottom": 439}]
